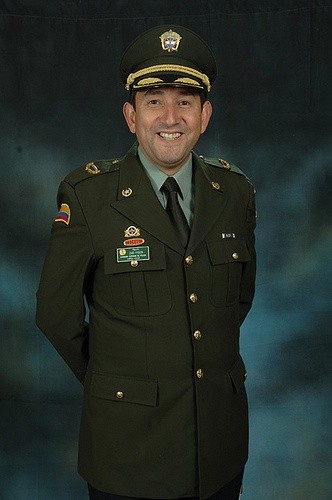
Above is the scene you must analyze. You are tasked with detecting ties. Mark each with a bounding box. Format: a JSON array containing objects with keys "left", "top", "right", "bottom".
[{"left": 160, "top": 177, "right": 192, "bottom": 251}]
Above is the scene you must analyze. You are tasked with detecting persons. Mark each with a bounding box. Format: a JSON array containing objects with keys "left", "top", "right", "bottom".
[{"left": 36, "top": 24, "right": 258, "bottom": 500}]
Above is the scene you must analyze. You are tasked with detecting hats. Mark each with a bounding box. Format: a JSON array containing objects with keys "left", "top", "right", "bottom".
[{"left": 119, "top": 24, "right": 218, "bottom": 102}]
